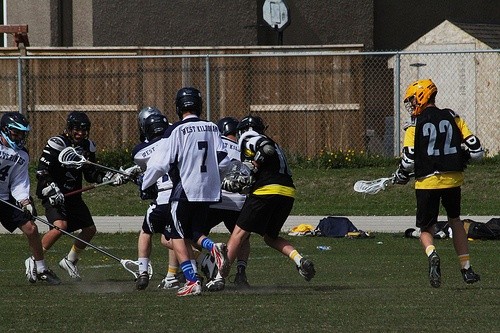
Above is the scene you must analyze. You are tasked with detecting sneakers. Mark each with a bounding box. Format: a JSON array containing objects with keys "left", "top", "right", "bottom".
[
  {"left": 25, "top": 257, "right": 37, "bottom": 283},
  {"left": 37, "top": 268, "right": 61, "bottom": 285},
  {"left": 58, "top": 258, "right": 81, "bottom": 279},
  {"left": 135, "top": 272, "right": 149, "bottom": 290},
  {"left": 296, "top": 258, "right": 315, "bottom": 282},
  {"left": 461, "top": 268, "right": 479, "bottom": 283},
  {"left": 174, "top": 242, "right": 249, "bottom": 296},
  {"left": 428, "top": 249, "right": 441, "bottom": 288},
  {"left": 157, "top": 278, "right": 179, "bottom": 289}
]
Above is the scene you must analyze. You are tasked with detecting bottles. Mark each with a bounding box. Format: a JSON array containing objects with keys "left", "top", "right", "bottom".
[{"left": 316, "top": 246, "right": 332, "bottom": 251}]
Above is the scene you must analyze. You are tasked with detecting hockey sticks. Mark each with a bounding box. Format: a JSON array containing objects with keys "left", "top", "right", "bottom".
[
  {"left": 64, "top": 164, "right": 146, "bottom": 197},
  {"left": 58, "top": 146, "right": 130, "bottom": 176},
  {"left": 0, "top": 198, "right": 153, "bottom": 280},
  {"left": 353, "top": 173, "right": 415, "bottom": 195}
]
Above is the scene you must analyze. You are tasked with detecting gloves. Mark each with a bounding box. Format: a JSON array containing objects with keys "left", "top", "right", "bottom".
[
  {"left": 243, "top": 160, "right": 257, "bottom": 172},
  {"left": 220, "top": 175, "right": 246, "bottom": 192},
  {"left": 140, "top": 183, "right": 158, "bottom": 200},
  {"left": 391, "top": 166, "right": 410, "bottom": 186},
  {"left": 102, "top": 172, "right": 128, "bottom": 186},
  {"left": 41, "top": 182, "right": 65, "bottom": 206},
  {"left": 18, "top": 196, "right": 37, "bottom": 221}
]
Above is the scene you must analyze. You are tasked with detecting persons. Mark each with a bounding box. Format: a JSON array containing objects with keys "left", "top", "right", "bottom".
[
  {"left": 201, "top": 115, "right": 316, "bottom": 291},
  {"left": 25, "top": 110, "right": 125, "bottom": 283},
  {"left": 383, "top": 79, "right": 482, "bottom": 288},
  {"left": 131, "top": 86, "right": 252, "bottom": 296},
  {"left": 0, "top": 111, "right": 62, "bottom": 285}
]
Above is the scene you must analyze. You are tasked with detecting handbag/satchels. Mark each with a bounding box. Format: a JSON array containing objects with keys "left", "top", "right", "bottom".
[
  {"left": 461, "top": 218, "right": 495, "bottom": 239},
  {"left": 318, "top": 215, "right": 357, "bottom": 237}
]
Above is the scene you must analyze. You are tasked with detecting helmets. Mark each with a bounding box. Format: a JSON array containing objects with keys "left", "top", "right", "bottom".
[
  {"left": 67, "top": 112, "right": 91, "bottom": 145},
  {"left": 237, "top": 115, "right": 265, "bottom": 132},
  {"left": 403, "top": 79, "right": 437, "bottom": 117},
  {"left": 217, "top": 116, "right": 239, "bottom": 137},
  {"left": 145, "top": 113, "right": 168, "bottom": 141},
  {"left": 138, "top": 107, "right": 161, "bottom": 134},
  {"left": 176, "top": 87, "right": 202, "bottom": 116},
  {"left": 0, "top": 112, "right": 32, "bottom": 152}
]
